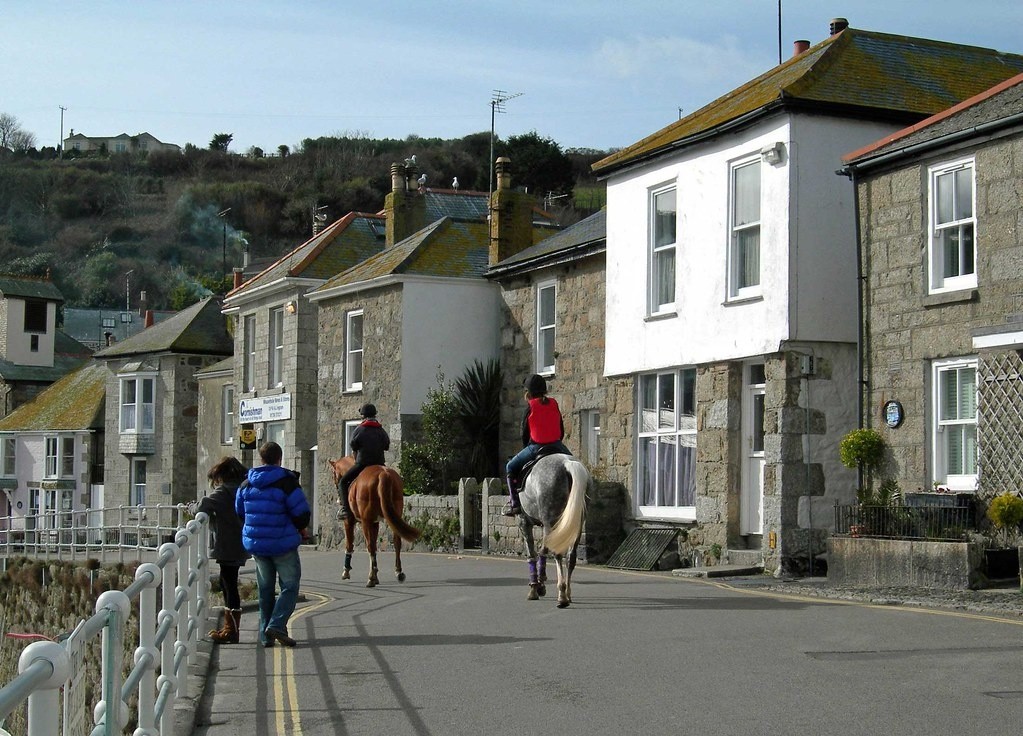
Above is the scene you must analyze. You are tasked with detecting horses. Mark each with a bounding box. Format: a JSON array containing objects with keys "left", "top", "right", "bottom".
[
  {"left": 506, "top": 453, "right": 596, "bottom": 609},
  {"left": 328, "top": 455, "right": 423, "bottom": 588}
]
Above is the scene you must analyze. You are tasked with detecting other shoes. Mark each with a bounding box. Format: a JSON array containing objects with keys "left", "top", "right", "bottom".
[
  {"left": 265, "top": 628, "right": 297, "bottom": 647},
  {"left": 263, "top": 638, "right": 276, "bottom": 647}
]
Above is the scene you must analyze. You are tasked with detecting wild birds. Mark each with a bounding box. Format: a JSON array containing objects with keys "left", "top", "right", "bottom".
[
  {"left": 452, "top": 176, "right": 459, "bottom": 191},
  {"left": 404, "top": 154, "right": 417, "bottom": 168},
  {"left": 417, "top": 174, "right": 427, "bottom": 188}
]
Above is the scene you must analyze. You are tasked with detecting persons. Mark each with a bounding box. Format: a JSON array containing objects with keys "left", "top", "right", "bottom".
[
  {"left": 502, "top": 373, "right": 575, "bottom": 513},
  {"left": 336, "top": 403, "right": 392, "bottom": 520},
  {"left": 195, "top": 456, "right": 254, "bottom": 642},
  {"left": 235, "top": 441, "right": 312, "bottom": 646}
]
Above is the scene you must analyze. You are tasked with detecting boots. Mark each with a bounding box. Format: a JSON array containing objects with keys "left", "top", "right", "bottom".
[
  {"left": 209, "top": 608, "right": 243, "bottom": 643},
  {"left": 503, "top": 477, "right": 521, "bottom": 513},
  {"left": 336, "top": 489, "right": 350, "bottom": 518},
  {"left": 208, "top": 628, "right": 226, "bottom": 636}
]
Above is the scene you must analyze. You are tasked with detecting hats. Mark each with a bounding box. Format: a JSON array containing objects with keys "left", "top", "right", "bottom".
[
  {"left": 358, "top": 404, "right": 377, "bottom": 415},
  {"left": 525, "top": 374, "right": 549, "bottom": 394}
]
